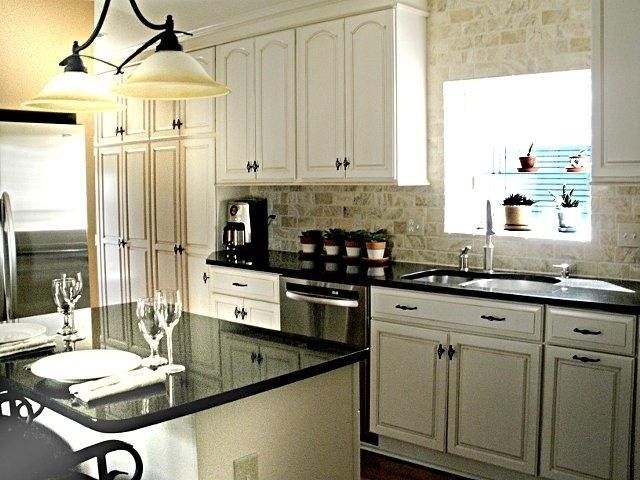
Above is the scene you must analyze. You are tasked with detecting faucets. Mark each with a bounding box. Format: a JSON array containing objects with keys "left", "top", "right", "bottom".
[{"left": 482, "top": 199, "right": 495, "bottom": 274}]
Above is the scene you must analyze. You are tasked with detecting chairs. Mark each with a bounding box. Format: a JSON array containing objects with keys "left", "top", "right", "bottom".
[{"left": 0, "top": 393, "right": 143, "bottom": 480}]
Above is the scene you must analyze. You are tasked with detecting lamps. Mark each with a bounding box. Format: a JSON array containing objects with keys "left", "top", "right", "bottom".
[{"left": 21, "top": 1, "right": 231, "bottom": 113}]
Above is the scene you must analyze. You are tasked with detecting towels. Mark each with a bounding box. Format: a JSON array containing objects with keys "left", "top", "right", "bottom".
[
  {"left": 0, "top": 334, "right": 56, "bottom": 357},
  {"left": 68, "top": 368, "right": 166, "bottom": 402}
]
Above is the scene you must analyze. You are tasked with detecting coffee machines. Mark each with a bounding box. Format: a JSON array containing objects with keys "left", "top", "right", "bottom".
[{"left": 223, "top": 200, "right": 268, "bottom": 259}]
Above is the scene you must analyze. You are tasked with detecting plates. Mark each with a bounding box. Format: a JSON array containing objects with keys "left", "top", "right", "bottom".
[
  {"left": 0, "top": 323, "right": 46, "bottom": 343},
  {"left": 30, "top": 349, "right": 142, "bottom": 383}
]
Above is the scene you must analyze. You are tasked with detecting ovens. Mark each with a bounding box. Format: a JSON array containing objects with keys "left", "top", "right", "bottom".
[{"left": 279, "top": 272, "right": 378, "bottom": 447}]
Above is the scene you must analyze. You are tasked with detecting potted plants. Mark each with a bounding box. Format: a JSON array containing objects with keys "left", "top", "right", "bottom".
[
  {"left": 548, "top": 183, "right": 581, "bottom": 232},
  {"left": 298, "top": 228, "right": 396, "bottom": 267},
  {"left": 502, "top": 194, "right": 539, "bottom": 231},
  {"left": 567, "top": 147, "right": 591, "bottom": 172},
  {"left": 516, "top": 143, "right": 538, "bottom": 173}
]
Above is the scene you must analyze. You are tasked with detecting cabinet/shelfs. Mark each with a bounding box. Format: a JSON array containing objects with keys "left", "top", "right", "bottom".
[
  {"left": 215, "top": 4, "right": 430, "bottom": 187},
  {"left": 209, "top": 263, "right": 281, "bottom": 331},
  {"left": 94, "top": 47, "right": 250, "bottom": 317},
  {"left": 592, "top": 1, "right": 640, "bottom": 182}
]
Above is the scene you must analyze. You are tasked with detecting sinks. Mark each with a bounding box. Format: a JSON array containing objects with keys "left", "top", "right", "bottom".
[
  {"left": 461, "top": 273, "right": 561, "bottom": 298},
  {"left": 401, "top": 269, "right": 476, "bottom": 291}
]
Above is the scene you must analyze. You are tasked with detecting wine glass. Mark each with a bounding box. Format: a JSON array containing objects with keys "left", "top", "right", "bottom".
[
  {"left": 52, "top": 278, "right": 77, "bottom": 335},
  {"left": 60, "top": 272, "right": 84, "bottom": 342},
  {"left": 154, "top": 290, "right": 185, "bottom": 373},
  {"left": 136, "top": 298, "right": 168, "bottom": 367}
]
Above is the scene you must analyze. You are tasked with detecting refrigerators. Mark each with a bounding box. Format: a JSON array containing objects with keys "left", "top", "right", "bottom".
[{"left": 0, "top": 122, "right": 90, "bottom": 319}]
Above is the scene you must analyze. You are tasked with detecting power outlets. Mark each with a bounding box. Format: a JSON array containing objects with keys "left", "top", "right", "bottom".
[{"left": 407, "top": 216, "right": 425, "bottom": 236}]
